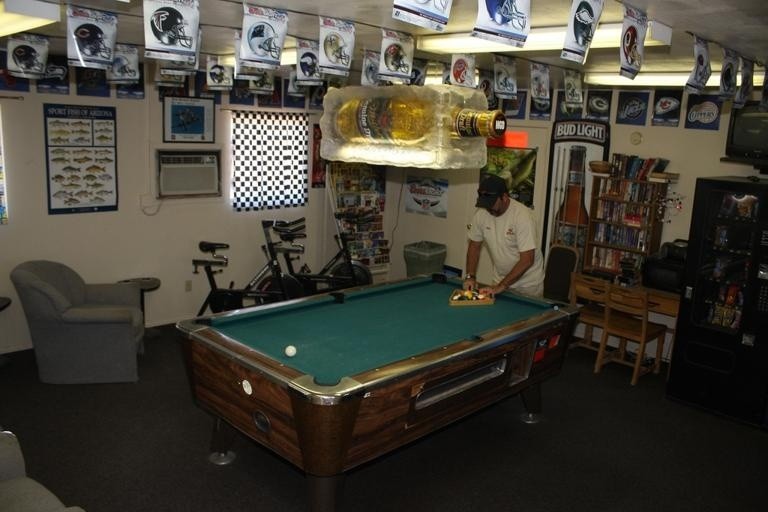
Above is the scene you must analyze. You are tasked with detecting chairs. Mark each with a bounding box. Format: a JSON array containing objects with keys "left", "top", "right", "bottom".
[
  {"left": 593, "top": 280, "right": 667, "bottom": 385},
  {"left": 543, "top": 244, "right": 580, "bottom": 308},
  {"left": 9, "top": 260, "right": 146, "bottom": 385},
  {"left": 570, "top": 273, "right": 612, "bottom": 352},
  {"left": 0, "top": 431, "right": 88, "bottom": 512}
]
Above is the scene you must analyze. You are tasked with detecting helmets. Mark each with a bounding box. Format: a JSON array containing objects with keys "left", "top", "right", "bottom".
[
  {"left": 485, "top": 1, "right": 525, "bottom": 32},
  {"left": 452, "top": 57, "right": 472, "bottom": 86},
  {"left": 694, "top": 47, "right": 752, "bottom": 100},
  {"left": 73, "top": 23, "right": 110, "bottom": 59},
  {"left": 149, "top": 6, "right": 192, "bottom": 47},
  {"left": 622, "top": 25, "right": 641, "bottom": 67},
  {"left": 13, "top": 44, "right": 43, "bottom": 72},
  {"left": 479, "top": 65, "right": 580, "bottom": 100},
  {"left": 573, "top": 0, "right": 594, "bottom": 47},
  {"left": 209, "top": 21, "right": 424, "bottom": 92},
  {"left": 110, "top": 54, "right": 136, "bottom": 76}
]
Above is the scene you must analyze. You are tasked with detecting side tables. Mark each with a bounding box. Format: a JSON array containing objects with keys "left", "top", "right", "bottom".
[{"left": 117, "top": 276, "right": 161, "bottom": 352}]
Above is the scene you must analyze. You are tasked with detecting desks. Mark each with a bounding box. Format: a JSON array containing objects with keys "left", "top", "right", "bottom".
[
  {"left": 569, "top": 271, "right": 682, "bottom": 382},
  {"left": 174, "top": 271, "right": 582, "bottom": 511}
]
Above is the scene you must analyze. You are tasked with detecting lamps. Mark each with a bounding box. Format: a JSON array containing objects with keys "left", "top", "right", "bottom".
[
  {"left": 0, "top": 0, "right": 60, "bottom": 38},
  {"left": 394, "top": 62, "right": 478, "bottom": 87},
  {"left": 415, "top": 21, "right": 673, "bottom": 55},
  {"left": 583, "top": 71, "right": 766, "bottom": 86},
  {"left": 218, "top": 46, "right": 298, "bottom": 65}
]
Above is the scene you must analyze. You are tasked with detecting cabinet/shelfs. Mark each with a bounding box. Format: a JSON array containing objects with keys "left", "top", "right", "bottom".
[
  {"left": 663, "top": 175, "right": 768, "bottom": 431},
  {"left": 583, "top": 174, "right": 668, "bottom": 282}
]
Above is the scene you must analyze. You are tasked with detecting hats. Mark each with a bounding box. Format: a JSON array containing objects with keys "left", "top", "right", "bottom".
[{"left": 475, "top": 175, "right": 505, "bottom": 208}]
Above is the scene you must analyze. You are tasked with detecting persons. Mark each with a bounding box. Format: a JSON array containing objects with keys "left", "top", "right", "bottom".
[{"left": 462, "top": 175, "right": 546, "bottom": 297}]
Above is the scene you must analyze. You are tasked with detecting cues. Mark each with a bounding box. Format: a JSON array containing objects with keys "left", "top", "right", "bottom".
[{"left": 550, "top": 146, "right": 586, "bottom": 247}]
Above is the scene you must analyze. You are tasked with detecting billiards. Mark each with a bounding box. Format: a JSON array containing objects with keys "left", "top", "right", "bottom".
[
  {"left": 285, "top": 345, "right": 296, "bottom": 356},
  {"left": 452, "top": 288, "right": 485, "bottom": 300}
]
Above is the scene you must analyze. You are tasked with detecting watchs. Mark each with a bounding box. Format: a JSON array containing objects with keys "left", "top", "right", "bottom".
[{"left": 465, "top": 275, "right": 476, "bottom": 280}]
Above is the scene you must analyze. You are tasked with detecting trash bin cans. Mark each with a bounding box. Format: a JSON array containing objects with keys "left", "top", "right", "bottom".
[{"left": 404, "top": 240, "right": 447, "bottom": 281}]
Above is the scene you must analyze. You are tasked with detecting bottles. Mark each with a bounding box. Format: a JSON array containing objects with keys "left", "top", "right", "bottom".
[
  {"left": 550, "top": 145, "right": 590, "bottom": 272},
  {"left": 331, "top": 98, "right": 506, "bottom": 148}
]
